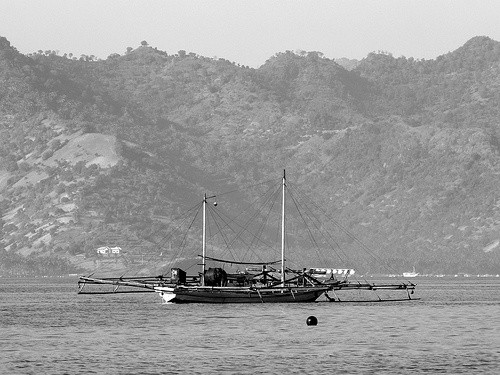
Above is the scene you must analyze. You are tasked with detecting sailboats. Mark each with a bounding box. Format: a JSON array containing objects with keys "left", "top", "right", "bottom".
[{"left": 71, "top": 168, "right": 419, "bottom": 302}]
